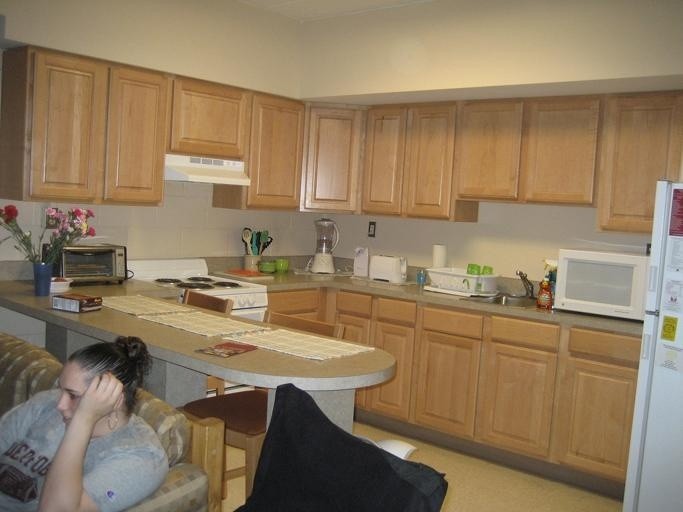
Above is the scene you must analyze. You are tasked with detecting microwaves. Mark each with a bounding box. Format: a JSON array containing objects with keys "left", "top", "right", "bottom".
[{"left": 554, "top": 247, "right": 650, "bottom": 323}]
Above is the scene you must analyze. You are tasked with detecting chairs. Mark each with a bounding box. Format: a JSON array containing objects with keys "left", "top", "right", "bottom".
[
  {"left": 180, "top": 289, "right": 234, "bottom": 396},
  {"left": 185, "top": 308, "right": 346, "bottom": 501}
]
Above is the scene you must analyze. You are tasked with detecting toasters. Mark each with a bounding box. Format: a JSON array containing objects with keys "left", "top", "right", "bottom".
[{"left": 369, "top": 251, "right": 407, "bottom": 286}]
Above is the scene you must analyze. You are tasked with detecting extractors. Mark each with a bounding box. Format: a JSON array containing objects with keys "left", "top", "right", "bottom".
[{"left": 163, "top": 154, "right": 251, "bottom": 186}]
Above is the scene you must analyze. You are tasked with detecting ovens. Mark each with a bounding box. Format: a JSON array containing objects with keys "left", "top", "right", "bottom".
[{"left": 40, "top": 243, "right": 135, "bottom": 285}]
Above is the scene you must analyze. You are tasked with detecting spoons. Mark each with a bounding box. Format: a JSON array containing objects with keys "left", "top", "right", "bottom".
[
  {"left": 259, "top": 229, "right": 269, "bottom": 255},
  {"left": 241, "top": 230, "right": 254, "bottom": 255}
]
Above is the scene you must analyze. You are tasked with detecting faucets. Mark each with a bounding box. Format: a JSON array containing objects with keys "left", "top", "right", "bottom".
[{"left": 515, "top": 269, "right": 534, "bottom": 296}]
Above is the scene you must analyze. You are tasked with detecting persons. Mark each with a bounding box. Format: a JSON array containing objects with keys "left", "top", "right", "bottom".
[{"left": 1, "top": 335, "right": 170, "bottom": 511}]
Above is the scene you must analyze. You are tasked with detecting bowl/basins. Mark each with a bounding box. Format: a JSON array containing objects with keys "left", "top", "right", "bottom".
[{"left": 259, "top": 261, "right": 277, "bottom": 275}]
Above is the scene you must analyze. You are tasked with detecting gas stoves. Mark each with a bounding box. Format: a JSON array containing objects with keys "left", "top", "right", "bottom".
[{"left": 126, "top": 259, "right": 270, "bottom": 309}]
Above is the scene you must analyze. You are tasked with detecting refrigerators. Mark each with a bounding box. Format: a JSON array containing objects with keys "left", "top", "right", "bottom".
[{"left": 620, "top": 178, "right": 683, "bottom": 511}]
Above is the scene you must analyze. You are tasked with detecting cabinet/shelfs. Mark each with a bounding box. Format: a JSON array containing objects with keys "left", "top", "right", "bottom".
[
  {"left": 331, "top": 288, "right": 416, "bottom": 430},
  {"left": 298, "top": 102, "right": 363, "bottom": 220},
  {"left": 551, "top": 326, "right": 642, "bottom": 484},
  {"left": 595, "top": 90, "right": 683, "bottom": 235},
  {"left": 413, "top": 304, "right": 561, "bottom": 460},
  {"left": 0, "top": 44, "right": 170, "bottom": 207},
  {"left": 453, "top": 96, "right": 600, "bottom": 207},
  {"left": 212, "top": 95, "right": 306, "bottom": 211},
  {"left": 360, "top": 101, "right": 479, "bottom": 223},
  {"left": 167, "top": 78, "right": 249, "bottom": 161}
]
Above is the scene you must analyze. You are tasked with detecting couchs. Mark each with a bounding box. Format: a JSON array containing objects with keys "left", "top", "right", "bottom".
[{"left": 1, "top": 329, "right": 225, "bottom": 511}]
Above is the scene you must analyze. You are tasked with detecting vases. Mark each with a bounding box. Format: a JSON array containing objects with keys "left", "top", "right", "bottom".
[{"left": 33, "top": 263, "right": 51, "bottom": 297}]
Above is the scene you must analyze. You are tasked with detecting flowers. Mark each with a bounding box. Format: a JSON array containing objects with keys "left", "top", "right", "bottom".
[{"left": 0, "top": 203, "right": 95, "bottom": 263}]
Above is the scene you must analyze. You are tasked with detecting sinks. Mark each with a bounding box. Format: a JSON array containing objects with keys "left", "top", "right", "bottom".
[{"left": 466, "top": 294, "right": 538, "bottom": 308}]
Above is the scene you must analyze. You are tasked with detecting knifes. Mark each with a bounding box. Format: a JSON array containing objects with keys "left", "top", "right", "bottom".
[{"left": 251, "top": 231, "right": 258, "bottom": 255}]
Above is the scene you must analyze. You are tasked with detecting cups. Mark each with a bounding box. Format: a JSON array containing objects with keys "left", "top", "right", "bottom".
[
  {"left": 276, "top": 258, "right": 289, "bottom": 272},
  {"left": 243, "top": 255, "right": 262, "bottom": 273}
]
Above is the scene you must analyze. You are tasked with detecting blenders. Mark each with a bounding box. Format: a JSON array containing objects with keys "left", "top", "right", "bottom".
[{"left": 310, "top": 218, "right": 338, "bottom": 274}]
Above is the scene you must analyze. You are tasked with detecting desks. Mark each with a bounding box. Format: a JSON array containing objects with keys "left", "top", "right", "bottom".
[{"left": 0, "top": 277, "right": 397, "bottom": 436}]
